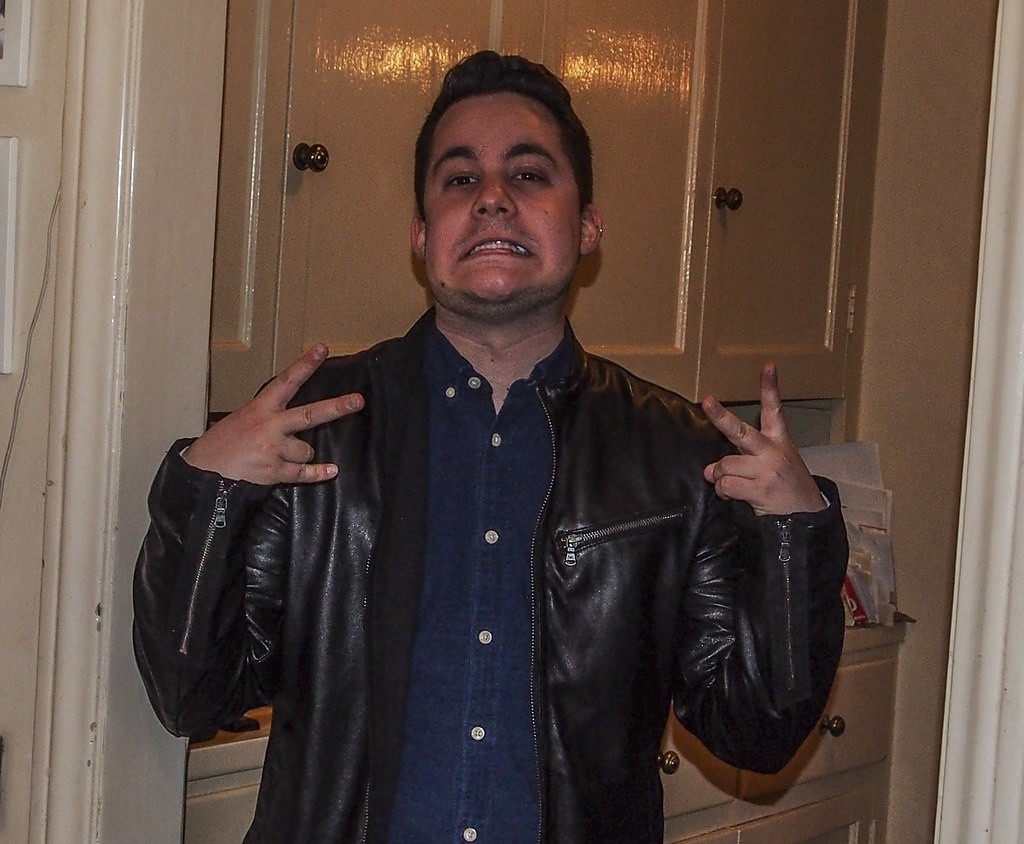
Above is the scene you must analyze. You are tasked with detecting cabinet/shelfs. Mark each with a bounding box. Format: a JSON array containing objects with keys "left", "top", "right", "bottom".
[
  {"left": 205, "top": 0, "right": 858, "bottom": 413},
  {"left": 183, "top": 657, "right": 893, "bottom": 844}
]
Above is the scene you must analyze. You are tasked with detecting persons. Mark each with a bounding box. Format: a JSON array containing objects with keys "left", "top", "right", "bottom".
[{"left": 133, "top": 51, "right": 849, "bottom": 844}]
